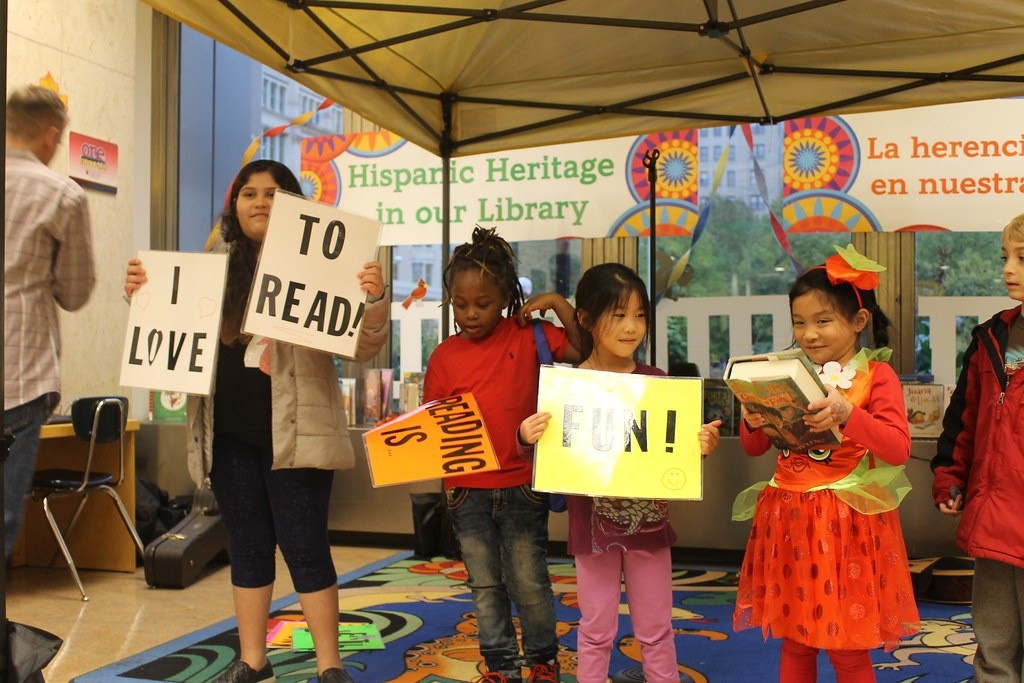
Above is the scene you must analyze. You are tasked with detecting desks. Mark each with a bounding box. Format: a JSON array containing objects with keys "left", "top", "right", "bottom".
[{"left": 10, "top": 420, "right": 140, "bottom": 573}]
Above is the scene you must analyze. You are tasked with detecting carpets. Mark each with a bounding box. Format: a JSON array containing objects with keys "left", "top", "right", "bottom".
[{"left": 64, "top": 551, "right": 978, "bottom": 683}]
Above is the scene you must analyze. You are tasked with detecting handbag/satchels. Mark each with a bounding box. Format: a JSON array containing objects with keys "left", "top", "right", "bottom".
[{"left": 533, "top": 318, "right": 568, "bottom": 512}]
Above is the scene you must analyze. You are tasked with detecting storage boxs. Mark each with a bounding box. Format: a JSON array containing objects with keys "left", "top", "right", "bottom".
[
  {"left": 903, "top": 383, "right": 955, "bottom": 438},
  {"left": 706, "top": 385, "right": 741, "bottom": 436}
]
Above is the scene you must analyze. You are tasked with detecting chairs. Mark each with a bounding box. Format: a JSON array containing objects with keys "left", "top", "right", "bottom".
[{"left": 33, "top": 397, "right": 144, "bottom": 601}]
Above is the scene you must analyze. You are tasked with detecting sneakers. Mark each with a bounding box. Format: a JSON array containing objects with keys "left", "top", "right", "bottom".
[
  {"left": 526, "top": 659, "right": 560, "bottom": 683},
  {"left": 316, "top": 667, "right": 357, "bottom": 683},
  {"left": 212, "top": 658, "right": 276, "bottom": 683},
  {"left": 469, "top": 659, "right": 522, "bottom": 683}
]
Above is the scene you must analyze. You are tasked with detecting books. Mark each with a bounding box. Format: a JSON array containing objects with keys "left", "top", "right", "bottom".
[{"left": 723, "top": 348, "right": 843, "bottom": 454}]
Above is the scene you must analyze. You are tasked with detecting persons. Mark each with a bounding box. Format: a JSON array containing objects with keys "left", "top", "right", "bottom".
[
  {"left": 516, "top": 262, "right": 722, "bottom": 683},
  {"left": 731, "top": 244, "right": 921, "bottom": 683},
  {"left": 375, "top": 223, "right": 590, "bottom": 683},
  {"left": 122, "top": 160, "right": 390, "bottom": 683},
  {"left": 3, "top": 85, "right": 97, "bottom": 579},
  {"left": 930, "top": 216, "right": 1024, "bottom": 683}
]
